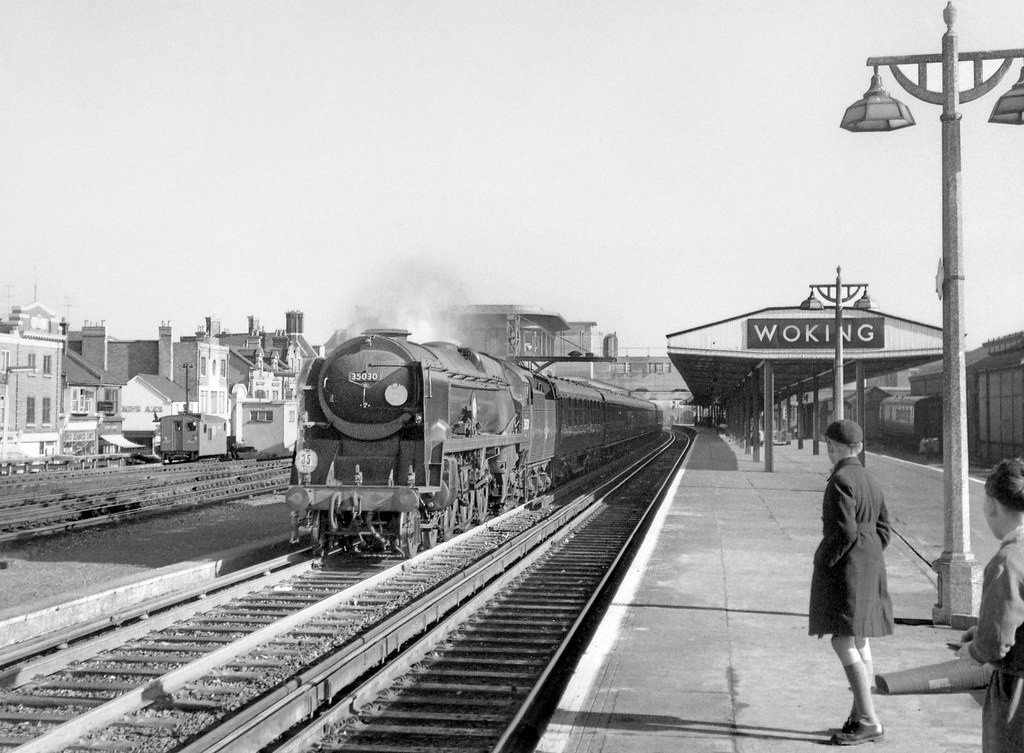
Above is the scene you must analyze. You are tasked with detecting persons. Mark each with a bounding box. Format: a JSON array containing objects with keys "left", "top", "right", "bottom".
[
  {"left": 808, "top": 420, "right": 895, "bottom": 746},
  {"left": 955, "top": 458, "right": 1023, "bottom": 753}
]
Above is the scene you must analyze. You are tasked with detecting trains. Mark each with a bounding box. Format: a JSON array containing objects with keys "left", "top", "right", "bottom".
[{"left": 285, "top": 327, "right": 663, "bottom": 565}]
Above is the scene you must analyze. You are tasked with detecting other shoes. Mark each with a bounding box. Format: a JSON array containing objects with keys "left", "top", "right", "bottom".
[{"left": 831, "top": 718, "right": 884, "bottom": 746}]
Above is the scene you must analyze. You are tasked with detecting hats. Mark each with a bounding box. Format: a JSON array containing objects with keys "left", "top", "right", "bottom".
[{"left": 825, "top": 419, "right": 863, "bottom": 443}]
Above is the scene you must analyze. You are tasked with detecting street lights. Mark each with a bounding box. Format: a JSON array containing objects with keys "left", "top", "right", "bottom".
[{"left": 840, "top": 0, "right": 1023, "bottom": 633}]
